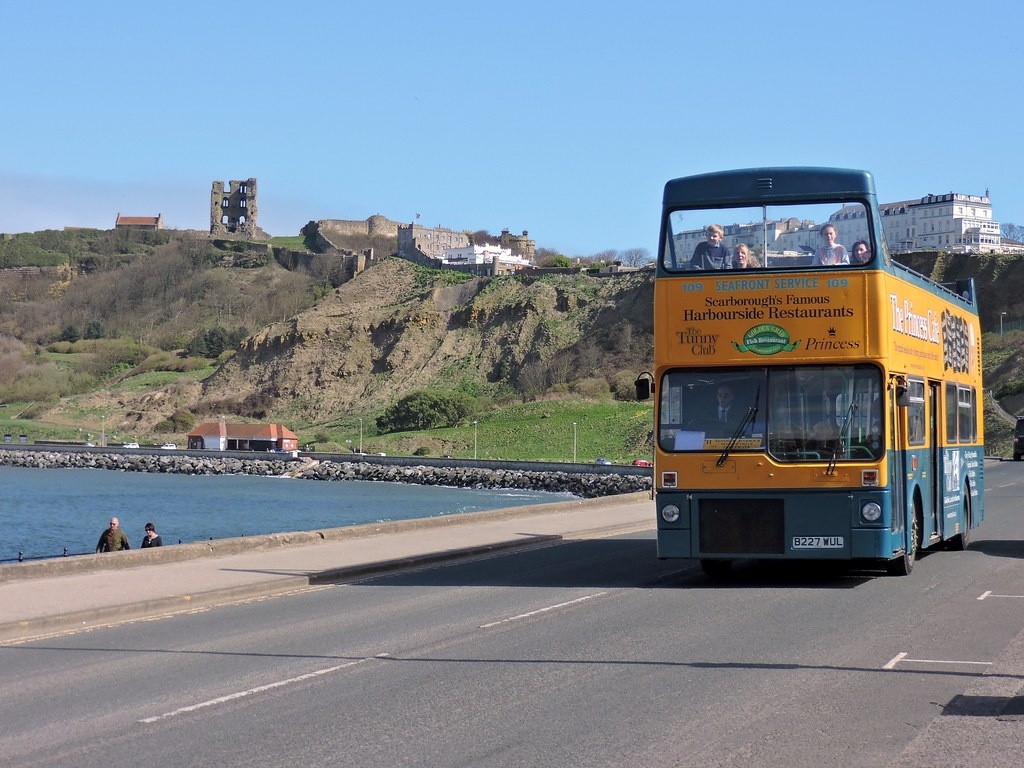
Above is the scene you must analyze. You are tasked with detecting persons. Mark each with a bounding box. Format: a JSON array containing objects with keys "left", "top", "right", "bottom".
[
  {"left": 811, "top": 223, "right": 850, "bottom": 265},
  {"left": 712, "top": 385, "right": 739, "bottom": 426},
  {"left": 852, "top": 240, "right": 871, "bottom": 263},
  {"left": 689, "top": 224, "right": 733, "bottom": 269},
  {"left": 733, "top": 242, "right": 761, "bottom": 268},
  {"left": 141, "top": 522, "right": 162, "bottom": 548},
  {"left": 96, "top": 517, "right": 131, "bottom": 553},
  {"left": 805, "top": 420, "right": 841, "bottom": 456}
]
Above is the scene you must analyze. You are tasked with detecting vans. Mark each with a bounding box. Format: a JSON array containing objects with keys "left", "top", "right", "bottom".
[
  {"left": 122, "top": 443, "right": 139, "bottom": 449},
  {"left": 161, "top": 444, "right": 177, "bottom": 449}
]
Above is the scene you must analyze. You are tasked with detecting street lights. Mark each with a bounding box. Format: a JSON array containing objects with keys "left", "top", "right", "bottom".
[
  {"left": 358, "top": 418, "right": 362, "bottom": 453},
  {"left": 572, "top": 422, "right": 576, "bottom": 463},
  {"left": 473, "top": 422, "right": 477, "bottom": 459},
  {"left": 102, "top": 416, "right": 104, "bottom": 447}
]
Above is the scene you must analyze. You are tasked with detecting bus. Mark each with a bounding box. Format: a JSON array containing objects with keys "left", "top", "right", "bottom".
[{"left": 634, "top": 166, "right": 985, "bottom": 575}]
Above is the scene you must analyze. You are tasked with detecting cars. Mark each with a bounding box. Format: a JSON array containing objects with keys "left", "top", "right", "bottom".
[
  {"left": 81, "top": 443, "right": 94, "bottom": 447},
  {"left": 440, "top": 454, "right": 454, "bottom": 459},
  {"left": 275, "top": 449, "right": 289, "bottom": 453},
  {"left": 595, "top": 458, "right": 611, "bottom": 465},
  {"left": 633, "top": 460, "right": 652, "bottom": 467}
]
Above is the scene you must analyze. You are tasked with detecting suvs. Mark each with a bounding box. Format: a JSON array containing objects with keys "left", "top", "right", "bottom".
[{"left": 1013, "top": 418, "right": 1024, "bottom": 461}]
